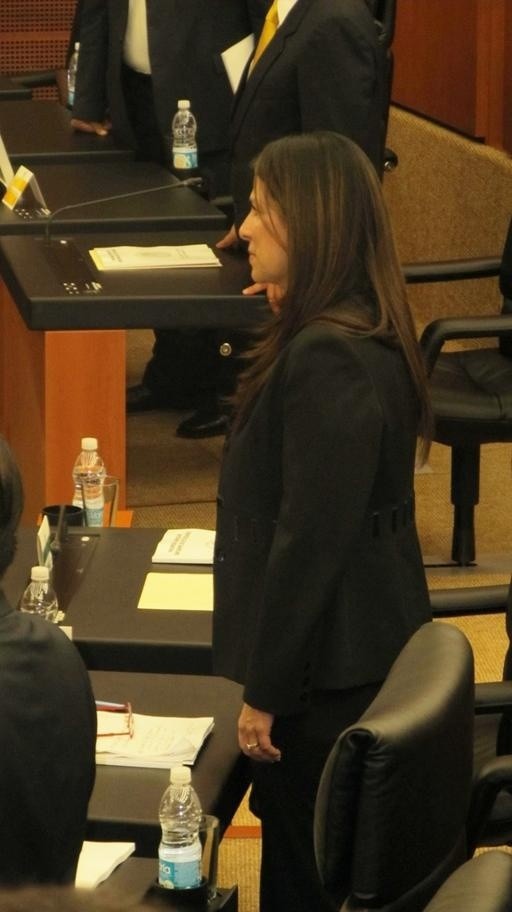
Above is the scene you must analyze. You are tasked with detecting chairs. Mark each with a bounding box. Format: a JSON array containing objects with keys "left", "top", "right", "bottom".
[{"left": 312, "top": 227, "right": 511, "bottom": 911}]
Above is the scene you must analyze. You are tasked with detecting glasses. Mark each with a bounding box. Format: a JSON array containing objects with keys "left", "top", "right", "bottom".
[{"left": 96, "top": 702, "right": 134, "bottom": 739}]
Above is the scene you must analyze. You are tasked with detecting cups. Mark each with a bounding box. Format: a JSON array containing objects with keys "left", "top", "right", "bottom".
[
  {"left": 190, "top": 813, "right": 222, "bottom": 903},
  {"left": 148, "top": 877, "right": 208, "bottom": 910},
  {"left": 41, "top": 504, "right": 84, "bottom": 526},
  {"left": 79, "top": 475, "right": 121, "bottom": 529}
]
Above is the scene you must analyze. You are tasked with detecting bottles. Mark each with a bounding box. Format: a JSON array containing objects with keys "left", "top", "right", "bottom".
[
  {"left": 172, "top": 100, "right": 199, "bottom": 169},
  {"left": 18, "top": 565, "right": 59, "bottom": 626},
  {"left": 67, "top": 42, "right": 80, "bottom": 106},
  {"left": 160, "top": 767, "right": 206, "bottom": 890},
  {"left": 71, "top": 436, "right": 105, "bottom": 526}
]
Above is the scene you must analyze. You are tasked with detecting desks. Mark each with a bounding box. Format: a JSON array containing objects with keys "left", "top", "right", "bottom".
[{"left": 1, "top": 96, "right": 278, "bottom": 910}]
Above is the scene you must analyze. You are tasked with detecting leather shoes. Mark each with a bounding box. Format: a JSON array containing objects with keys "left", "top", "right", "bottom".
[
  {"left": 126, "top": 384, "right": 195, "bottom": 411},
  {"left": 174, "top": 406, "right": 232, "bottom": 437}
]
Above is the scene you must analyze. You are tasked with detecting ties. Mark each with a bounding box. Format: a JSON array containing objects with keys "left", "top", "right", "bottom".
[{"left": 247, "top": 1, "right": 278, "bottom": 81}]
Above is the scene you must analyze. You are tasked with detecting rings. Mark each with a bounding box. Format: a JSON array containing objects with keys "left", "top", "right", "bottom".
[{"left": 246, "top": 743, "right": 258, "bottom": 749}]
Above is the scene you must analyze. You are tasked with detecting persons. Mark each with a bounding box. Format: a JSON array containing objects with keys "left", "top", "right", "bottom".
[
  {"left": 65, "top": 0, "right": 264, "bottom": 413},
  {"left": 177, "top": 0, "right": 397, "bottom": 436},
  {"left": 211, "top": 126, "right": 435, "bottom": 911},
  {"left": 0, "top": 439, "right": 98, "bottom": 888}
]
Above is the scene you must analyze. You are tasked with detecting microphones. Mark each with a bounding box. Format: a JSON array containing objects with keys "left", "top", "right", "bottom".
[{"left": 44, "top": 176, "right": 204, "bottom": 239}]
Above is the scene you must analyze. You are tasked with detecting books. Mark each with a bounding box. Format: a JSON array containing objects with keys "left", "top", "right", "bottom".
[
  {"left": 153, "top": 526, "right": 218, "bottom": 567},
  {"left": 88, "top": 240, "right": 224, "bottom": 270},
  {"left": 93, "top": 706, "right": 218, "bottom": 772},
  {"left": 76, "top": 838, "right": 135, "bottom": 892},
  {"left": 138, "top": 570, "right": 216, "bottom": 611}
]
